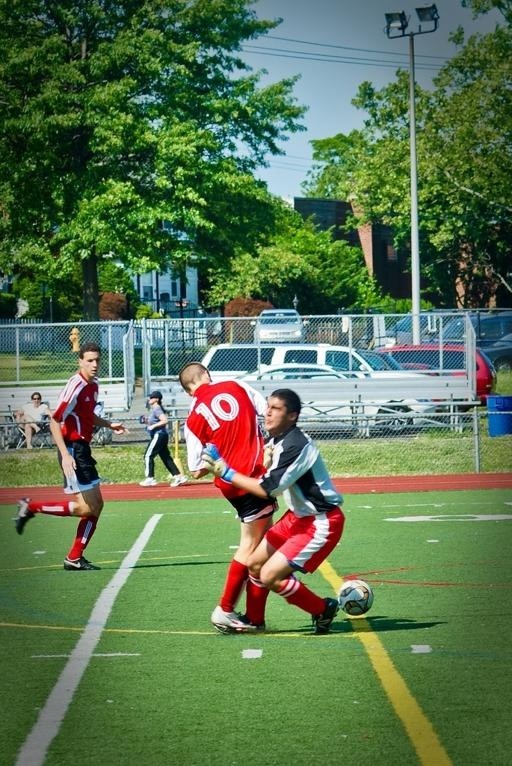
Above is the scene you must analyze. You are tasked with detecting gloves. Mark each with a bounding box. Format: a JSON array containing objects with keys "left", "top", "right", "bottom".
[{"left": 201, "top": 443, "right": 236, "bottom": 481}]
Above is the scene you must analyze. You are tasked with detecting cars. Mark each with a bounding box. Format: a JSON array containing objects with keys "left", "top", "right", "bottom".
[
  {"left": 198, "top": 362, "right": 439, "bottom": 434},
  {"left": 250, "top": 309, "right": 311, "bottom": 346}
]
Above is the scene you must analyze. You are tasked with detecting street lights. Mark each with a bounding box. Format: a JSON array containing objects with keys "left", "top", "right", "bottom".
[{"left": 384, "top": 3, "right": 445, "bottom": 340}]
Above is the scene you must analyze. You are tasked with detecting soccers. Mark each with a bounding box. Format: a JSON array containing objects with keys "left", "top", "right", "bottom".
[{"left": 339, "top": 580, "right": 372, "bottom": 616}]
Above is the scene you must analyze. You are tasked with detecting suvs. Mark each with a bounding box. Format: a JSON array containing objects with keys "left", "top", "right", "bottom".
[{"left": 373, "top": 343, "right": 495, "bottom": 419}]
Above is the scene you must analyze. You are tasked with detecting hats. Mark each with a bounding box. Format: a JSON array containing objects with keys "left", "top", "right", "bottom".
[{"left": 147, "top": 391, "right": 162, "bottom": 398}]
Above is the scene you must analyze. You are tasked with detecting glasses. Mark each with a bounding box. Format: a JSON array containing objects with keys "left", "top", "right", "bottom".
[{"left": 32, "top": 398, "right": 41, "bottom": 400}]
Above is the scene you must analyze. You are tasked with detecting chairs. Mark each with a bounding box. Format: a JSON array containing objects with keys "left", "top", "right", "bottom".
[{"left": 18, "top": 401, "right": 54, "bottom": 450}]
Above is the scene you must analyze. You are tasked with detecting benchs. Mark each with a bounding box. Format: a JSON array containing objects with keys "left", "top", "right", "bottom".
[{"left": 0, "top": 377, "right": 131, "bottom": 417}]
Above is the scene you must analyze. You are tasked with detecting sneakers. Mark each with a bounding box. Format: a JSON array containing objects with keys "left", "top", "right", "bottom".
[
  {"left": 139, "top": 478, "right": 158, "bottom": 487},
  {"left": 170, "top": 476, "right": 188, "bottom": 487},
  {"left": 214, "top": 614, "right": 265, "bottom": 633},
  {"left": 313, "top": 598, "right": 340, "bottom": 632},
  {"left": 15, "top": 498, "right": 35, "bottom": 535},
  {"left": 64, "top": 556, "right": 101, "bottom": 570},
  {"left": 211, "top": 605, "right": 257, "bottom": 634}
]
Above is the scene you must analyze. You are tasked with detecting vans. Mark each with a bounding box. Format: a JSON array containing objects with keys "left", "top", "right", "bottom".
[
  {"left": 199, "top": 343, "right": 436, "bottom": 430},
  {"left": 359, "top": 312, "right": 511, "bottom": 368}
]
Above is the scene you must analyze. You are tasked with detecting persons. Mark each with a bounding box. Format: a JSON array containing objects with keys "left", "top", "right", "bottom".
[
  {"left": 178, "top": 362, "right": 279, "bottom": 635},
  {"left": 13, "top": 391, "right": 53, "bottom": 450},
  {"left": 138, "top": 390, "right": 188, "bottom": 488},
  {"left": 14, "top": 341, "right": 131, "bottom": 571},
  {"left": 198, "top": 387, "right": 346, "bottom": 634}
]
